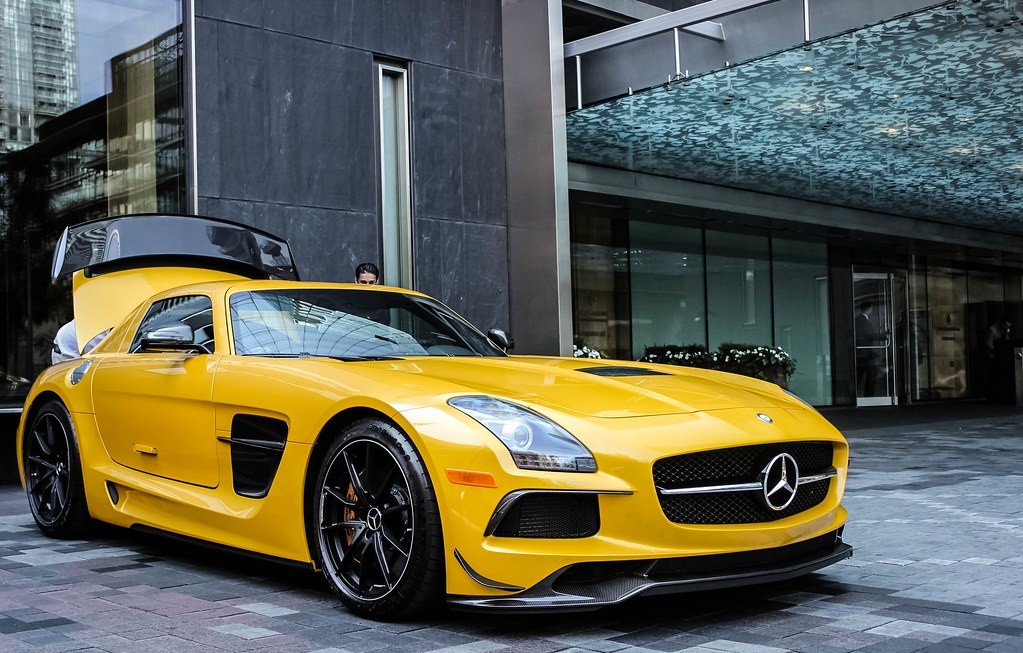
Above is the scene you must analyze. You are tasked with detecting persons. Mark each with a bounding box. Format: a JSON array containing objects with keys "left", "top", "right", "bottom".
[
  {"left": 52, "top": 318, "right": 113, "bottom": 364},
  {"left": 347, "top": 263, "right": 391, "bottom": 327}
]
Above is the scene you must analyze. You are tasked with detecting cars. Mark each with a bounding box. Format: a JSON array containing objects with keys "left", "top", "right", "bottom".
[{"left": 0, "top": 368, "right": 33, "bottom": 473}]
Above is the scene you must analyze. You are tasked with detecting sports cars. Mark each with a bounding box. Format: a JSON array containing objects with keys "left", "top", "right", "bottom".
[{"left": 16, "top": 212, "right": 852, "bottom": 624}]
